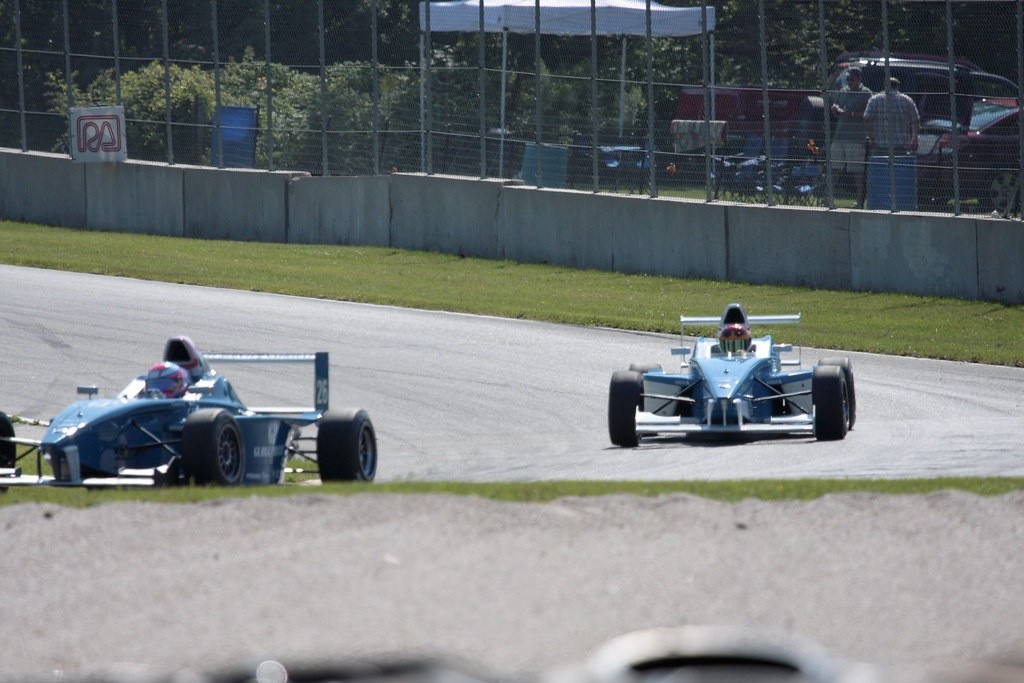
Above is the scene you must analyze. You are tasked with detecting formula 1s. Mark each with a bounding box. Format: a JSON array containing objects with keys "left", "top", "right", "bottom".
[
  {"left": 606, "top": 302, "right": 856, "bottom": 451},
  {"left": 0, "top": 337, "right": 378, "bottom": 495}
]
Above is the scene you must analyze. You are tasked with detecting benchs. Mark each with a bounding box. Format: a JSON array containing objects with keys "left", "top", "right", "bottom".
[{"left": 566, "top": 135, "right": 676, "bottom": 194}]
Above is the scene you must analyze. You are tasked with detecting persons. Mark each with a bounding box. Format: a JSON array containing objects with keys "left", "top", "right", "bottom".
[
  {"left": 821, "top": 67, "right": 872, "bottom": 209},
  {"left": 719, "top": 325, "right": 751, "bottom": 352},
  {"left": 864, "top": 77, "right": 919, "bottom": 155},
  {"left": 146, "top": 363, "right": 190, "bottom": 399}
]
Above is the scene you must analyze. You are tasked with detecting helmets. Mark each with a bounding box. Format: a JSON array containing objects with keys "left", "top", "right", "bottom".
[
  {"left": 145, "top": 360, "right": 188, "bottom": 399},
  {"left": 719, "top": 324, "right": 751, "bottom": 353}
]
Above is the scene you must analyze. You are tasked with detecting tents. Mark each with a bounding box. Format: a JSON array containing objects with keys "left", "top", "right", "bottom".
[{"left": 419, "top": 0, "right": 715, "bottom": 191}]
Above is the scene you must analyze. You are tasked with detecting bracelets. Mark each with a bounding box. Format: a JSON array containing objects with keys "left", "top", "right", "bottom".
[{"left": 851, "top": 111, "right": 854, "bottom": 117}]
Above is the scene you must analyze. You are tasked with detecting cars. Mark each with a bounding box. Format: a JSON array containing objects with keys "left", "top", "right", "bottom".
[{"left": 917, "top": 94, "right": 1023, "bottom": 215}]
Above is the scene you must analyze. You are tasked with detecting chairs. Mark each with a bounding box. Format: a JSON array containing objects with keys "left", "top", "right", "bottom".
[
  {"left": 708, "top": 154, "right": 831, "bottom": 207},
  {"left": 598, "top": 143, "right": 669, "bottom": 195}
]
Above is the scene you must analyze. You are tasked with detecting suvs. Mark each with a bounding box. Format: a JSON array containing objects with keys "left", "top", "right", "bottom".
[{"left": 825, "top": 56, "right": 1021, "bottom": 128}]
